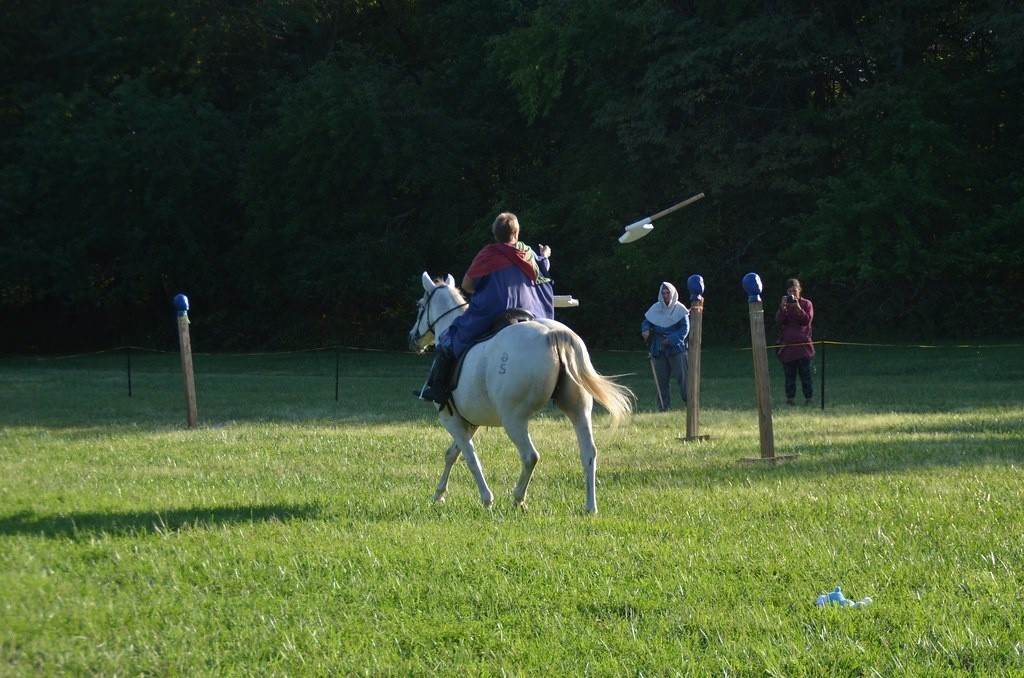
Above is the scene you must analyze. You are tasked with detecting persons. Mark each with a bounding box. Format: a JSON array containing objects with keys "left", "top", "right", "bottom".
[
  {"left": 413, "top": 211, "right": 554, "bottom": 405},
  {"left": 641, "top": 282, "right": 690, "bottom": 410},
  {"left": 775, "top": 279, "right": 816, "bottom": 407}
]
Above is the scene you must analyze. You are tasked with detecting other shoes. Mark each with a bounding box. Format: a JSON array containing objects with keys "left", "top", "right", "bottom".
[
  {"left": 806, "top": 400, "right": 814, "bottom": 407},
  {"left": 786, "top": 401, "right": 794, "bottom": 406}
]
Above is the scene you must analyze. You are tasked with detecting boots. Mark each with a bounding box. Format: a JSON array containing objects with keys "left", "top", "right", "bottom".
[{"left": 413, "top": 373, "right": 455, "bottom": 405}]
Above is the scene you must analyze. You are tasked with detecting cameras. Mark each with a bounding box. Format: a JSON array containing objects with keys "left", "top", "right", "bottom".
[{"left": 786, "top": 295, "right": 797, "bottom": 303}]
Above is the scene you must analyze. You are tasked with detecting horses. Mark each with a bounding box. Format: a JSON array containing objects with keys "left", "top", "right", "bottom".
[{"left": 410, "top": 269, "right": 638, "bottom": 517}]
